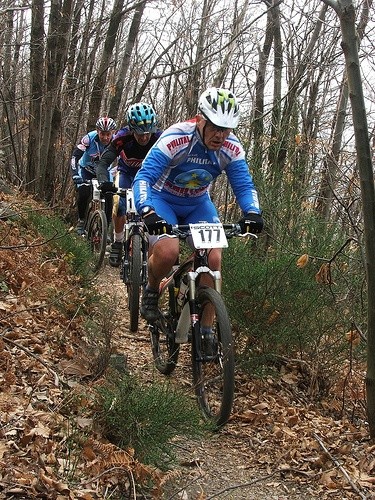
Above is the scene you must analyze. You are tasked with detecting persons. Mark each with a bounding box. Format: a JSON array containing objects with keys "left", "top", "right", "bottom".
[
  {"left": 130, "top": 86, "right": 263, "bottom": 359},
  {"left": 96, "top": 102, "right": 164, "bottom": 268},
  {"left": 72, "top": 117, "right": 120, "bottom": 243}
]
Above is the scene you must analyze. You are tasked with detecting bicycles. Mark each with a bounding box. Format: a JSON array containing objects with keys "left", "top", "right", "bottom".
[
  {"left": 141, "top": 218, "right": 259, "bottom": 433},
  {"left": 81, "top": 179, "right": 149, "bottom": 333}
]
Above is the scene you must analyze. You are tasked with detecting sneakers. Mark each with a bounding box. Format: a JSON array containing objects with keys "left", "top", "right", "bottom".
[
  {"left": 76, "top": 222, "right": 84, "bottom": 235},
  {"left": 98, "top": 233, "right": 111, "bottom": 243},
  {"left": 109, "top": 240, "right": 123, "bottom": 267},
  {"left": 140, "top": 285, "right": 158, "bottom": 319},
  {"left": 199, "top": 333, "right": 218, "bottom": 364}
]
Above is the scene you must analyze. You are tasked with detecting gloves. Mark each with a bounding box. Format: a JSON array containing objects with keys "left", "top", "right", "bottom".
[
  {"left": 101, "top": 181, "right": 118, "bottom": 195},
  {"left": 142, "top": 212, "right": 172, "bottom": 235},
  {"left": 73, "top": 178, "right": 82, "bottom": 184},
  {"left": 237, "top": 212, "right": 264, "bottom": 236}
]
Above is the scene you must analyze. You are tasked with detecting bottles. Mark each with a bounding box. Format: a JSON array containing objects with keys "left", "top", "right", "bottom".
[{"left": 176, "top": 274, "right": 190, "bottom": 307}]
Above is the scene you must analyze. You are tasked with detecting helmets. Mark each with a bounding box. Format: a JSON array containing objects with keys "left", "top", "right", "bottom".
[
  {"left": 95, "top": 117, "right": 117, "bottom": 132},
  {"left": 198, "top": 87, "right": 240, "bottom": 128},
  {"left": 126, "top": 103, "right": 158, "bottom": 133}
]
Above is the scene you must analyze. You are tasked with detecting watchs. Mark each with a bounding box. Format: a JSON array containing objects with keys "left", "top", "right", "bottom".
[{"left": 141, "top": 206, "right": 154, "bottom": 216}]
